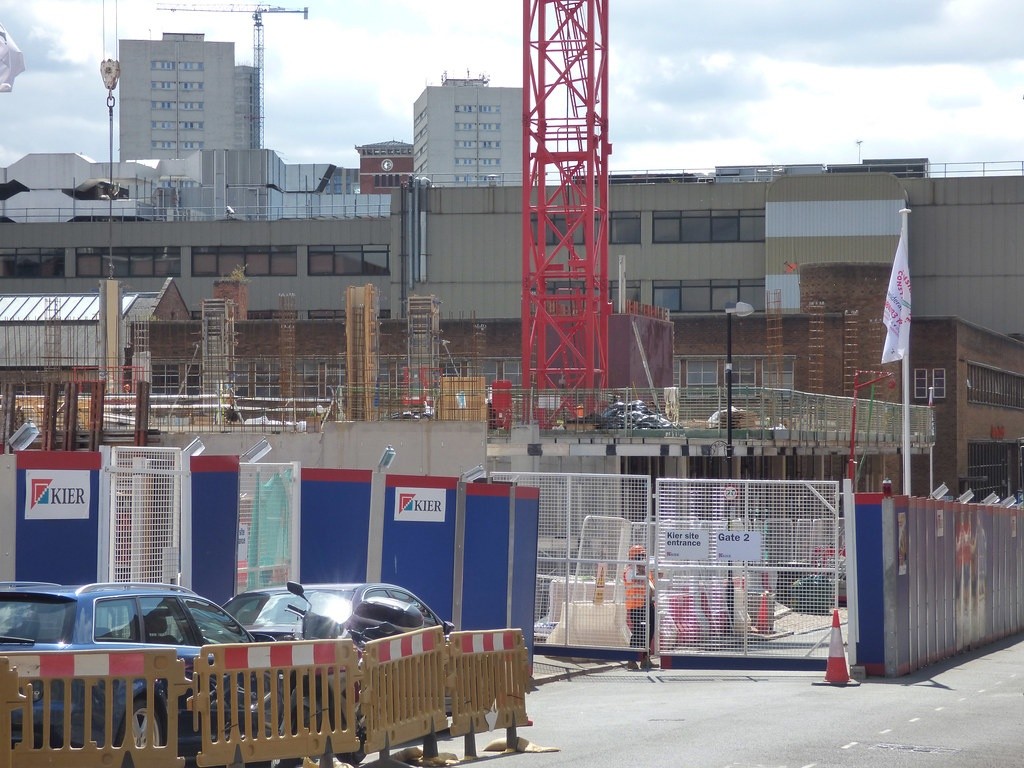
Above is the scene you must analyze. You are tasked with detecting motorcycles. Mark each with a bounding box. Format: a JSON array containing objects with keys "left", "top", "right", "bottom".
[{"left": 271, "top": 580, "right": 424, "bottom": 768}]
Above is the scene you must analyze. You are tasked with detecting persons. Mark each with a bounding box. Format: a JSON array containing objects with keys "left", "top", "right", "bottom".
[{"left": 624, "top": 545, "right": 659, "bottom": 669}]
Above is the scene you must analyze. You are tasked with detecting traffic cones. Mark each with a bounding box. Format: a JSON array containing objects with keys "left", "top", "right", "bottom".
[{"left": 811, "top": 609, "right": 861, "bottom": 686}]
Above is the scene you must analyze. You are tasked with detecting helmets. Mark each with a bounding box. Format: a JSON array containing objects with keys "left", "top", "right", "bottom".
[{"left": 628, "top": 546, "right": 645, "bottom": 559}]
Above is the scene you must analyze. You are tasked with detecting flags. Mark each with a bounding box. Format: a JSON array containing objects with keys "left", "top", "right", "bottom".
[{"left": 881, "top": 228, "right": 912, "bottom": 364}]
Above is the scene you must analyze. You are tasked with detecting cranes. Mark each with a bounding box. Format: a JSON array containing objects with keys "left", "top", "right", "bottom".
[{"left": 157, "top": 2, "right": 307, "bottom": 148}]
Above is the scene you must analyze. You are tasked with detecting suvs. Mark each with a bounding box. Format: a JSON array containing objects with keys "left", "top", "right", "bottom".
[{"left": 0, "top": 581, "right": 282, "bottom": 768}]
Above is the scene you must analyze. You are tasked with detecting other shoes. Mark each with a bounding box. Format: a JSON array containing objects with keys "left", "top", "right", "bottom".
[
  {"left": 628, "top": 660, "right": 639, "bottom": 670},
  {"left": 641, "top": 657, "right": 659, "bottom": 669}
]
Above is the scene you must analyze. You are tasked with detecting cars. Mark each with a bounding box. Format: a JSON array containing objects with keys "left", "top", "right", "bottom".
[{"left": 200, "top": 583, "right": 456, "bottom": 726}]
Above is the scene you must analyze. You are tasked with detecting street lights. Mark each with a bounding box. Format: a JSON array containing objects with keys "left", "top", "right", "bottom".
[
  {"left": 849, "top": 370, "right": 896, "bottom": 493},
  {"left": 855, "top": 139, "right": 863, "bottom": 162},
  {"left": 722, "top": 301, "right": 755, "bottom": 649}
]
